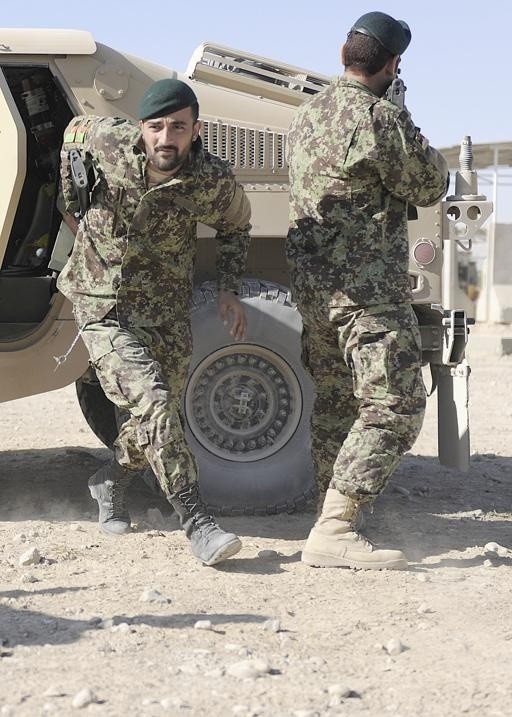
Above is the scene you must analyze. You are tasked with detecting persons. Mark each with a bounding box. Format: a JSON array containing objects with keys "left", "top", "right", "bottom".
[
  {"left": 287, "top": 11, "right": 450, "bottom": 572},
  {"left": 55, "top": 77, "right": 252, "bottom": 566},
  {"left": 2, "top": 64, "right": 74, "bottom": 267}
]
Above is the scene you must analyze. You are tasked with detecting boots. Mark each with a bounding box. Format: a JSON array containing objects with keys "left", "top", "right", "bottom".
[
  {"left": 166, "top": 482, "right": 242, "bottom": 566},
  {"left": 87, "top": 457, "right": 142, "bottom": 535},
  {"left": 301, "top": 487, "right": 408, "bottom": 571}
]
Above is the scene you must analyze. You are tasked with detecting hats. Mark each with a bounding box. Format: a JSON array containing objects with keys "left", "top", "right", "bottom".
[
  {"left": 347, "top": 12, "right": 412, "bottom": 56},
  {"left": 139, "top": 79, "right": 196, "bottom": 121}
]
